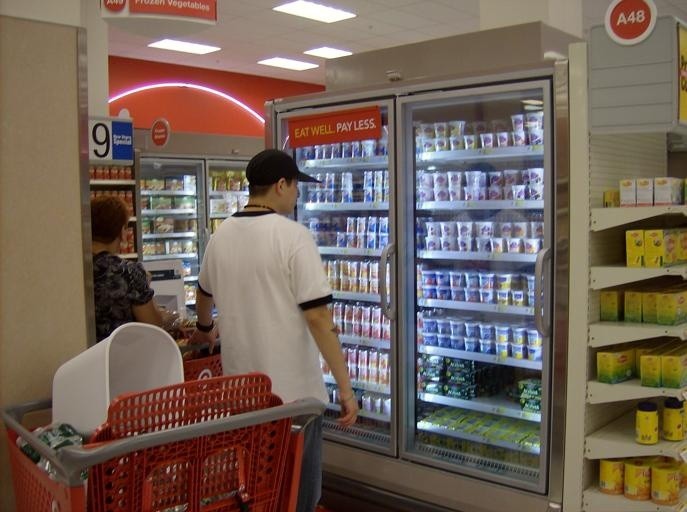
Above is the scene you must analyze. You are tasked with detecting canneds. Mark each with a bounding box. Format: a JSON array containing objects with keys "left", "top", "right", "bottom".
[
  {"left": 325, "top": 301, "right": 390, "bottom": 340},
  {"left": 319, "top": 343, "right": 391, "bottom": 416},
  {"left": 307, "top": 170, "right": 389, "bottom": 203},
  {"left": 302, "top": 216, "right": 388, "bottom": 250},
  {"left": 320, "top": 258, "right": 390, "bottom": 295}
]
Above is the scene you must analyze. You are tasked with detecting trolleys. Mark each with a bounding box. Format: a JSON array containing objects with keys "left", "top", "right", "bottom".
[{"left": 2, "top": 348, "right": 326, "bottom": 512}]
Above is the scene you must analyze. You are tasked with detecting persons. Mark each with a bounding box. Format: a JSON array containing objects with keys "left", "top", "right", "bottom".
[
  {"left": 89, "top": 194, "right": 178, "bottom": 345},
  {"left": 186, "top": 148, "right": 361, "bottom": 512}
]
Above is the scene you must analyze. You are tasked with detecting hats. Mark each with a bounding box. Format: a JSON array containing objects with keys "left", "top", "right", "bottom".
[{"left": 245, "top": 149, "right": 322, "bottom": 183}]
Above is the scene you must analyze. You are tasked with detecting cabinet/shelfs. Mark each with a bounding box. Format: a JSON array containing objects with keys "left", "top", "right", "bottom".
[
  {"left": 567, "top": 41, "right": 686, "bottom": 509},
  {"left": 90, "top": 149, "right": 144, "bottom": 267}
]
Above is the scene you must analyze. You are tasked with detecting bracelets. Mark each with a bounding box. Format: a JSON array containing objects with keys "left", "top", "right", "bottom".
[
  {"left": 194, "top": 319, "right": 214, "bottom": 334},
  {"left": 340, "top": 389, "right": 355, "bottom": 403}
]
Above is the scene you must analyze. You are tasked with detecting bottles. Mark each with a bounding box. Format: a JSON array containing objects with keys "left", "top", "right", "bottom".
[
  {"left": 88, "top": 164, "right": 134, "bottom": 255},
  {"left": 635, "top": 396, "right": 687, "bottom": 447}
]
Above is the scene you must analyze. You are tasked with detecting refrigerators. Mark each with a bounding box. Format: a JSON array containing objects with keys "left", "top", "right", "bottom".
[
  {"left": 137, "top": 149, "right": 266, "bottom": 327},
  {"left": 253, "top": 58, "right": 580, "bottom": 511}
]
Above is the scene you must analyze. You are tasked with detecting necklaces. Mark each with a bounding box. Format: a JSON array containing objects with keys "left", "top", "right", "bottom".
[{"left": 243, "top": 204, "right": 276, "bottom": 213}]
